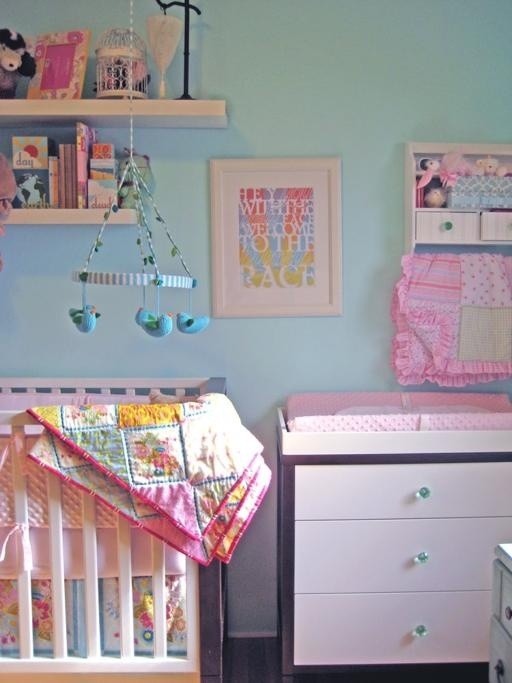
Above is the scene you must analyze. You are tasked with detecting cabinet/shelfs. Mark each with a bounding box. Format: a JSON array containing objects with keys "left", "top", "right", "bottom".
[
  {"left": 279, "top": 459, "right": 512, "bottom": 683},
  {"left": 0, "top": 96, "right": 231, "bottom": 228}
]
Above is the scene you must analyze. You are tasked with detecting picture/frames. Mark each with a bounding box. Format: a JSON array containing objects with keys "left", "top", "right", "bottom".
[{"left": 210, "top": 156, "right": 343, "bottom": 318}]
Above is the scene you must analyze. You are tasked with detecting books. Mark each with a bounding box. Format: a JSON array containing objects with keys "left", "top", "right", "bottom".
[{"left": 8, "top": 122, "right": 120, "bottom": 209}]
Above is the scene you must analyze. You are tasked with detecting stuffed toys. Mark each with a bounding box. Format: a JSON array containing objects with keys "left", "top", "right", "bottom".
[
  {"left": 424, "top": 187, "right": 449, "bottom": 207},
  {"left": 69, "top": 306, "right": 80, "bottom": 316},
  {"left": 1, "top": 27, "right": 36, "bottom": 99},
  {"left": 416, "top": 149, "right": 472, "bottom": 189},
  {"left": 134, "top": 306, "right": 155, "bottom": 325},
  {"left": 141, "top": 311, "right": 173, "bottom": 337},
  {"left": 114, "top": 146, "right": 154, "bottom": 208},
  {"left": 475, "top": 156, "right": 508, "bottom": 177},
  {"left": 176, "top": 311, "right": 210, "bottom": 333},
  {"left": 71, "top": 303, "right": 97, "bottom": 332}
]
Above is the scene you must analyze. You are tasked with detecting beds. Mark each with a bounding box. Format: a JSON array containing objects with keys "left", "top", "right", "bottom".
[{"left": 0, "top": 381, "right": 228, "bottom": 681}]
